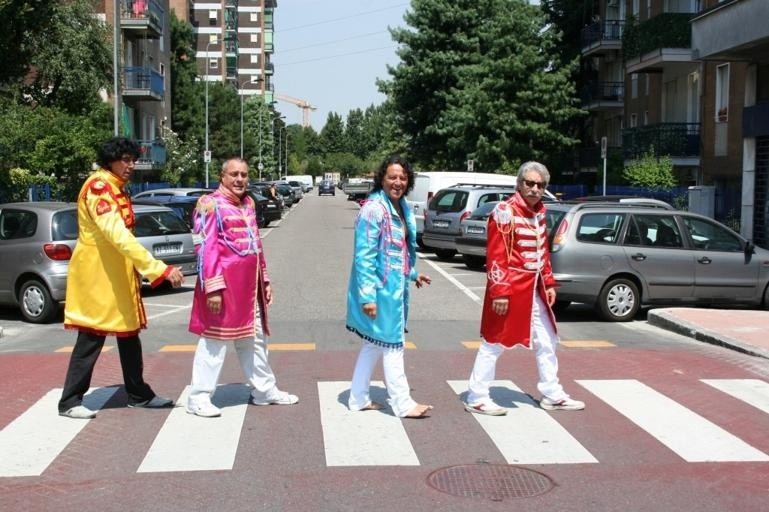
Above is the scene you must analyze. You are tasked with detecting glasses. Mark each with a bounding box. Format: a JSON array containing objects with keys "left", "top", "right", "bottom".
[{"left": 523, "top": 178, "right": 543, "bottom": 189}]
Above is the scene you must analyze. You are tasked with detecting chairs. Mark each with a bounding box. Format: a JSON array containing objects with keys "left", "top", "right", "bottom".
[
  {"left": 653, "top": 225, "right": 683, "bottom": 247},
  {"left": 3, "top": 217, "right": 27, "bottom": 240},
  {"left": 484, "top": 194, "right": 498, "bottom": 203},
  {"left": 461, "top": 199, "right": 466, "bottom": 209},
  {"left": 625, "top": 220, "right": 652, "bottom": 245}
]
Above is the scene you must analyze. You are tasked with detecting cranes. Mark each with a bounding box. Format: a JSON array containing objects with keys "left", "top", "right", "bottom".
[{"left": 275, "top": 91, "right": 316, "bottom": 128}]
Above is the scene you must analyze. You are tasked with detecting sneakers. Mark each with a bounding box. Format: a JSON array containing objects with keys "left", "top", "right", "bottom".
[
  {"left": 464, "top": 399, "right": 509, "bottom": 417},
  {"left": 538, "top": 394, "right": 585, "bottom": 412},
  {"left": 184, "top": 393, "right": 222, "bottom": 419},
  {"left": 58, "top": 402, "right": 97, "bottom": 420},
  {"left": 248, "top": 386, "right": 300, "bottom": 407},
  {"left": 126, "top": 392, "right": 174, "bottom": 409}
]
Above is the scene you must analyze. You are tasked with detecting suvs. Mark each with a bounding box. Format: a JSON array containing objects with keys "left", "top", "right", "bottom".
[
  {"left": 534, "top": 188, "right": 769, "bottom": 325},
  {"left": 338, "top": 168, "right": 570, "bottom": 272}
]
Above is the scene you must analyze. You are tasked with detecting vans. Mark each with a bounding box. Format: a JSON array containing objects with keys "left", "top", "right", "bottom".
[{"left": 317, "top": 180, "right": 335, "bottom": 197}]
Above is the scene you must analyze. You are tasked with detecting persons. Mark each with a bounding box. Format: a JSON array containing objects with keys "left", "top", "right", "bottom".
[
  {"left": 55, "top": 134, "right": 185, "bottom": 421},
  {"left": 458, "top": 161, "right": 588, "bottom": 417},
  {"left": 345, "top": 152, "right": 436, "bottom": 420},
  {"left": 182, "top": 154, "right": 299, "bottom": 419}
]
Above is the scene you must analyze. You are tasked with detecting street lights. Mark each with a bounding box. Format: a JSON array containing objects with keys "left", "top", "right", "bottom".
[
  {"left": 204, "top": 34, "right": 240, "bottom": 190},
  {"left": 240, "top": 79, "right": 264, "bottom": 160},
  {"left": 258, "top": 98, "right": 294, "bottom": 182}
]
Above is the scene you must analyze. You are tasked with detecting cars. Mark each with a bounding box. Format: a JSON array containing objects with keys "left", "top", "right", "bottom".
[
  {"left": 114, "top": 170, "right": 314, "bottom": 289},
  {"left": 0, "top": 198, "right": 79, "bottom": 325}
]
